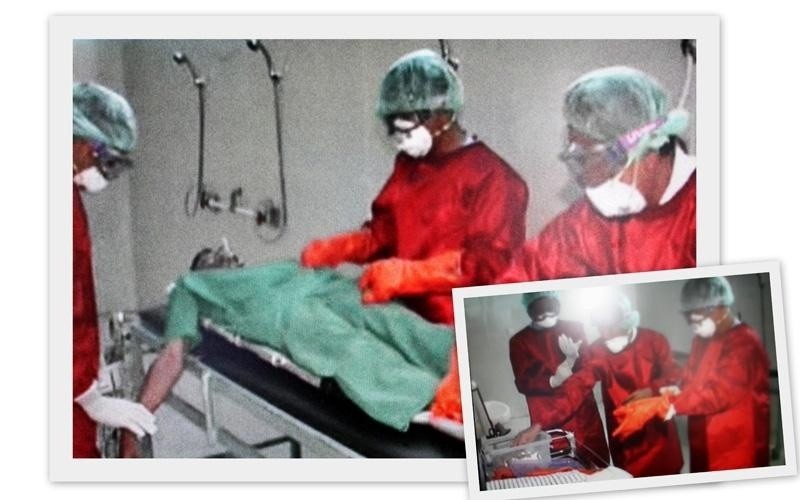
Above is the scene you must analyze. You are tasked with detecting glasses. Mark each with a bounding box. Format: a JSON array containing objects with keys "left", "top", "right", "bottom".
[
  {"left": 560, "top": 116, "right": 666, "bottom": 176},
  {"left": 381, "top": 109, "right": 440, "bottom": 136},
  {"left": 682, "top": 306, "right": 715, "bottom": 324},
  {"left": 526, "top": 298, "right": 559, "bottom": 321},
  {"left": 84, "top": 137, "right": 134, "bottom": 178}
]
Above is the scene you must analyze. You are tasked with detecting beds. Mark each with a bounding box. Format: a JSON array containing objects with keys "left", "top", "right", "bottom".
[{"left": 103, "top": 304, "right": 467, "bottom": 458}]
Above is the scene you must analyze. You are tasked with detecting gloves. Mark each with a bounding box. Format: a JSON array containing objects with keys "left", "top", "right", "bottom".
[
  {"left": 549, "top": 333, "right": 583, "bottom": 388},
  {"left": 302, "top": 229, "right": 377, "bottom": 269},
  {"left": 359, "top": 250, "right": 464, "bottom": 303},
  {"left": 74, "top": 380, "right": 158, "bottom": 440},
  {"left": 612, "top": 390, "right": 672, "bottom": 443},
  {"left": 432, "top": 346, "right": 464, "bottom": 424}
]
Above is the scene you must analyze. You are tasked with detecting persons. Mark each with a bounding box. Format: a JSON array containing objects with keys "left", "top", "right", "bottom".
[
  {"left": 119, "top": 248, "right": 455, "bottom": 459},
  {"left": 614, "top": 277, "right": 771, "bottom": 473},
  {"left": 73, "top": 80, "right": 157, "bottom": 458},
  {"left": 432, "top": 66, "right": 696, "bottom": 423},
  {"left": 509, "top": 293, "right": 610, "bottom": 466},
  {"left": 512, "top": 293, "right": 683, "bottom": 478},
  {"left": 301, "top": 47, "right": 528, "bottom": 326}
]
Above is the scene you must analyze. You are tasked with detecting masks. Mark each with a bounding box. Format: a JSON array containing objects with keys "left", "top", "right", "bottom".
[
  {"left": 73, "top": 164, "right": 108, "bottom": 195},
  {"left": 389, "top": 117, "right": 433, "bottom": 159},
  {"left": 689, "top": 314, "right": 716, "bottom": 339},
  {"left": 583, "top": 154, "right": 648, "bottom": 218},
  {"left": 605, "top": 336, "right": 628, "bottom": 354},
  {"left": 535, "top": 311, "right": 557, "bottom": 329}
]
